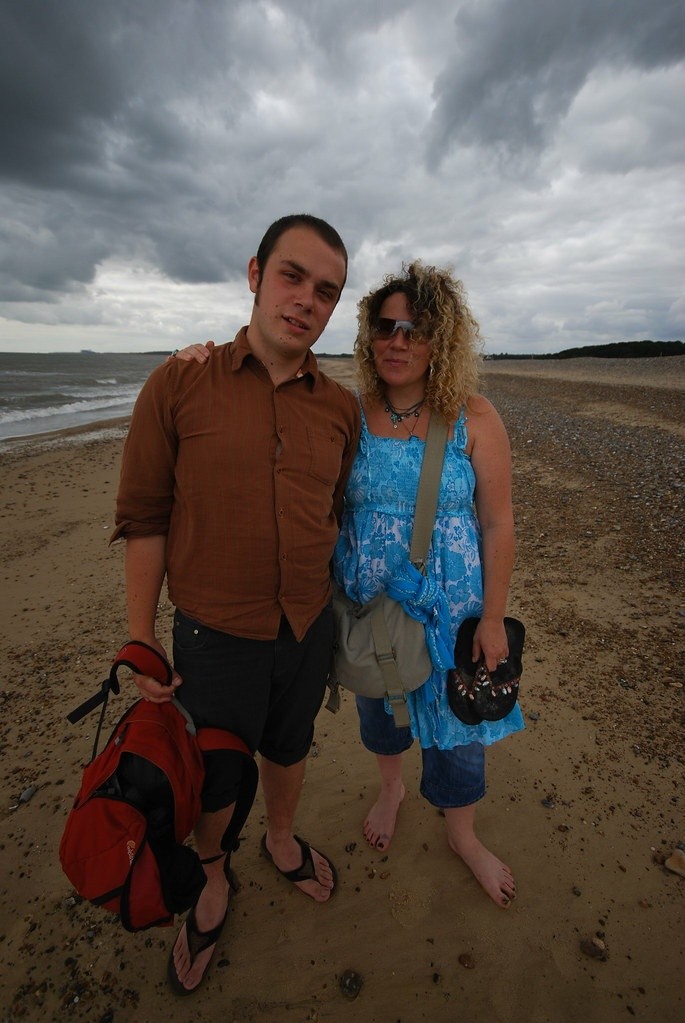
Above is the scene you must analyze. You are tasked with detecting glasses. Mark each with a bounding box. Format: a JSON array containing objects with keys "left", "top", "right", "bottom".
[{"left": 370, "top": 318, "right": 418, "bottom": 344}]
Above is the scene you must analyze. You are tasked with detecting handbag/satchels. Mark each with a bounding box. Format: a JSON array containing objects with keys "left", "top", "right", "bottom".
[{"left": 325, "top": 564, "right": 433, "bottom": 727}]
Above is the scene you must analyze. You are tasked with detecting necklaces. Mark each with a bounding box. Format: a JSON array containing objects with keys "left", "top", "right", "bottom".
[{"left": 384, "top": 388, "right": 426, "bottom": 441}]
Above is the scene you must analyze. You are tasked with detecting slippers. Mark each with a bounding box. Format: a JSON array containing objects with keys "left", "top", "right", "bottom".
[
  {"left": 474, "top": 617, "right": 526, "bottom": 722},
  {"left": 446, "top": 617, "right": 484, "bottom": 726},
  {"left": 167, "top": 898, "right": 229, "bottom": 996},
  {"left": 261, "top": 830, "right": 338, "bottom": 903}
]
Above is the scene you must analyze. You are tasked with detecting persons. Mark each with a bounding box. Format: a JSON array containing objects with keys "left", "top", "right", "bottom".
[
  {"left": 165, "top": 260, "right": 523, "bottom": 905},
  {"left": 110, "top": 213, "right": 363, "bottom": 997}
]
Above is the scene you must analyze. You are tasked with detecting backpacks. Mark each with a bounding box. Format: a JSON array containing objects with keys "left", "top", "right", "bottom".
[{"left": 60, "top": 641, "right": 259, "bottom": 933}]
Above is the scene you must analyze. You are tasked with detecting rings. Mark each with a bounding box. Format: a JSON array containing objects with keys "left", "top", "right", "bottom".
[
  {"left": 500, "top": 658, "right": 507, "bottom": 663},
  {"left": 172, "top": 349, "right": 180, "bottom": 358}
]
